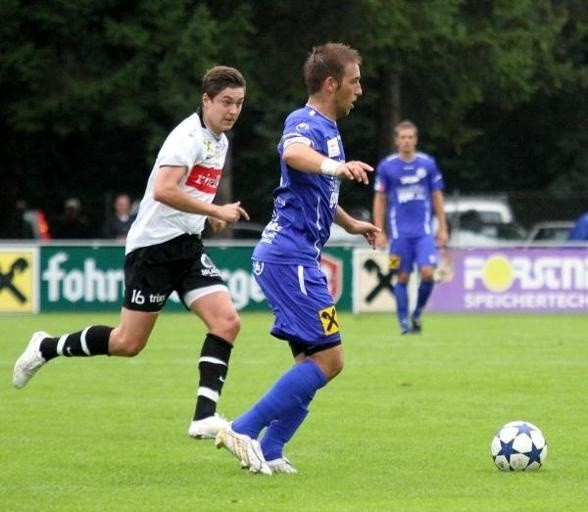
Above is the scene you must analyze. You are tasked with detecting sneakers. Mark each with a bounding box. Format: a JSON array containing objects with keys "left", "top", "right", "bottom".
[
  {"left": 214, "top": 436, "right": 272, "bottom": 475},
  {"left": 264, "top": 456, "right": 299, "bottom": 474},
  {"left": 411, "top": 316, "right": 422, "bottom": 333},
  {"left": 186, "top": 411, "right": 230, "bottom": 440},
  {"left": 398, "top": 319, "right": 410, "bottom": 335},
  {"left": 11, "top": 330, "right": 55, "bottom": 390}
]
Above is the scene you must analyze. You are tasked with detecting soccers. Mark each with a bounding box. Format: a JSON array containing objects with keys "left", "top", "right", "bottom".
[{"left": 489, "top": 421, "right": 545, "bottom": 472}]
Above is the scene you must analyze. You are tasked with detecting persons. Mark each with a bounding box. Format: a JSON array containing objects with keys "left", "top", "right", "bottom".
[
  {"left": 12, "top": 67, "right": 248, "bottom": 437},
  {"left": 0, "top": 194, "right": 138, "bottom": 239},
  {"left": 374, "top": 120, "right": 447, "bottom": 333},
  {"left": 214, "top": 42, "right": 382, "bottom": 477}
]
